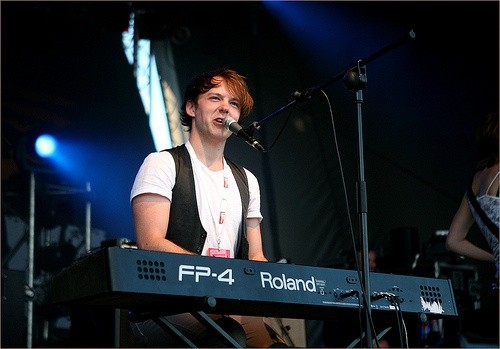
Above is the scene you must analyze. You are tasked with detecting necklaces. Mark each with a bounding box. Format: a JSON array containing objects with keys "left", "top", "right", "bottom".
[{"left": 194, "top": 154, "right": 230, "bottom": 252}]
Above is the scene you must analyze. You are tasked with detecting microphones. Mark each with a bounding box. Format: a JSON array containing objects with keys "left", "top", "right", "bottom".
[{"left": 222, "top": 116, "right": 264, "bottom": 151}]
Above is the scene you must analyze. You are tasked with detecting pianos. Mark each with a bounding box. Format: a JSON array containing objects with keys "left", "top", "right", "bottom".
[{"left": 63, "top": 247, "right": 461, "bottom": 349}]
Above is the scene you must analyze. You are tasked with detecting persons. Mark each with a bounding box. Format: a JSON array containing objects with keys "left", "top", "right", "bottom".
[
  {"left": 330, "top": 240, "right": 397, "bottom": 349},
  {"left": 444, "top": 115, "right": 500, "bottom": 349},
  {"left": 131, "top": 67, "right": 275, "bottom": 349}
]
